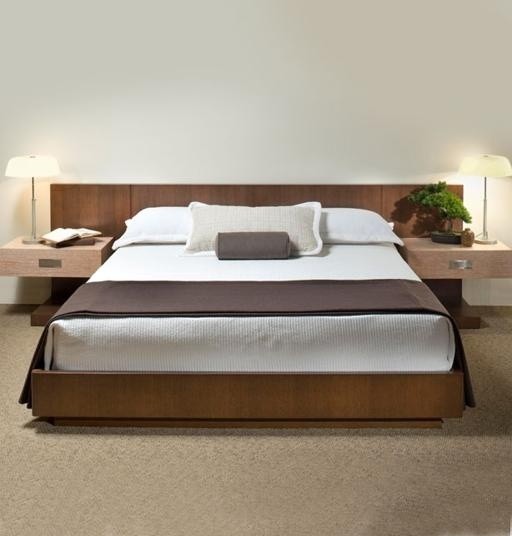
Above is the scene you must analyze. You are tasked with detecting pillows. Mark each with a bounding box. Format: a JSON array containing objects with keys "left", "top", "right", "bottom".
[
  {"left": 319, "top": 208, "right": 404, "bottom": 246},
  {"left": 214, "top": 231, "right": 291, "bottom": 260},
  {"left": 112, "top": 206, "right": 188, "bottom": 249},
  {"left": 185, "top": 201, "right": 323, "bottom": 256}
]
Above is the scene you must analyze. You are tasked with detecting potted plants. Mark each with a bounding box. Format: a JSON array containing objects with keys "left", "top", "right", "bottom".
[{"left": 407, "top": 180, "right": 472, "bottom": 244}]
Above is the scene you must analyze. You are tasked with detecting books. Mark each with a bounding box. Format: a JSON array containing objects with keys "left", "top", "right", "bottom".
[
  {"left": 65, "top": 238, "right": 96, "bottom": 247},
  {"left": 42, "top": 226, "right": 103, "bottom": 249}
]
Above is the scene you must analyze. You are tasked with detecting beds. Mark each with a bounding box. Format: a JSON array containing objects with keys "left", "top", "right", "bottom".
[{"left": 0, "top": 183, "right": 512, "bottom": 428}]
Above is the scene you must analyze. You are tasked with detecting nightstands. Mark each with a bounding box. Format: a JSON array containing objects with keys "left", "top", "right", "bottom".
[
  {"left": 395, "top": 237, "right": 512, "bottom": 329},
  {"left": 0, "top": 235, "right": 112, "bottom": 327}
]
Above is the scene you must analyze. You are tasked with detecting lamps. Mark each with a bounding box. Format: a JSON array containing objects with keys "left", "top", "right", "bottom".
[
  {"left": 459, "top": 154, "right": 512, "bottom": 244},
  {"left": 4, "top": 155, "right": 60, "bottom": 245}
]
